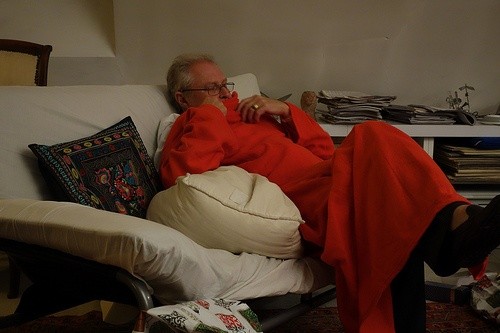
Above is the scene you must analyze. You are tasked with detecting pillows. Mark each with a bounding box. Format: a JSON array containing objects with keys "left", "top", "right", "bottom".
[
  {"left": 145, "top": 164, "right": 306, "bottom": 259},
  {"left": 28, "top": 114, "right": 166, "bottom": 220},
  {"left": 154, "top": 112, "right": 180, "bottom": 173},
  {"left": 144, "top": 297, "right": 264, "bottom": 333}
]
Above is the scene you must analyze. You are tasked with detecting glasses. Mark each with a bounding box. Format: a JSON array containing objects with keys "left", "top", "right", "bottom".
[{"left": 180, "top": 82, "right": 235, "bottom": 96}]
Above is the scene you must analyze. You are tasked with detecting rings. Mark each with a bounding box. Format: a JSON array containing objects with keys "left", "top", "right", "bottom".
[{"left": 252, "top": 104, "right": 259, "bottom": 110}]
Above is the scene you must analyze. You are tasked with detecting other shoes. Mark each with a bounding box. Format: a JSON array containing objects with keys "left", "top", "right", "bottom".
[{"left": 447, "top": 194, "right": 500, "bottom": 269}]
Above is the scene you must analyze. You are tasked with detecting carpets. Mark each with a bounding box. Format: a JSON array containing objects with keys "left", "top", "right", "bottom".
[{"left": 0, "top": 301, "right": 500, "bottom": 333}]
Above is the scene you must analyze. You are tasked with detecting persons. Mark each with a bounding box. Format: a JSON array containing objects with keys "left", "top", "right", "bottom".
[{"left": 157, "top": 53, "right": 500, "bottom": 333}]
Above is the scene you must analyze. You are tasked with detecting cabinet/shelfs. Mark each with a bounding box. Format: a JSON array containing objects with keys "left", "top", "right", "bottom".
[{"left": 314, "top": 113, "right": 500, "bottom": 305}]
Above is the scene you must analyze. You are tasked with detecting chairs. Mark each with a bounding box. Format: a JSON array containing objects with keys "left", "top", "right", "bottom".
[
  {"left": 0, "top": 72, "right": 338, "bottom": 333},
  {"left": 0, "top": 38, "right": 53, "bottom": 87}
]
